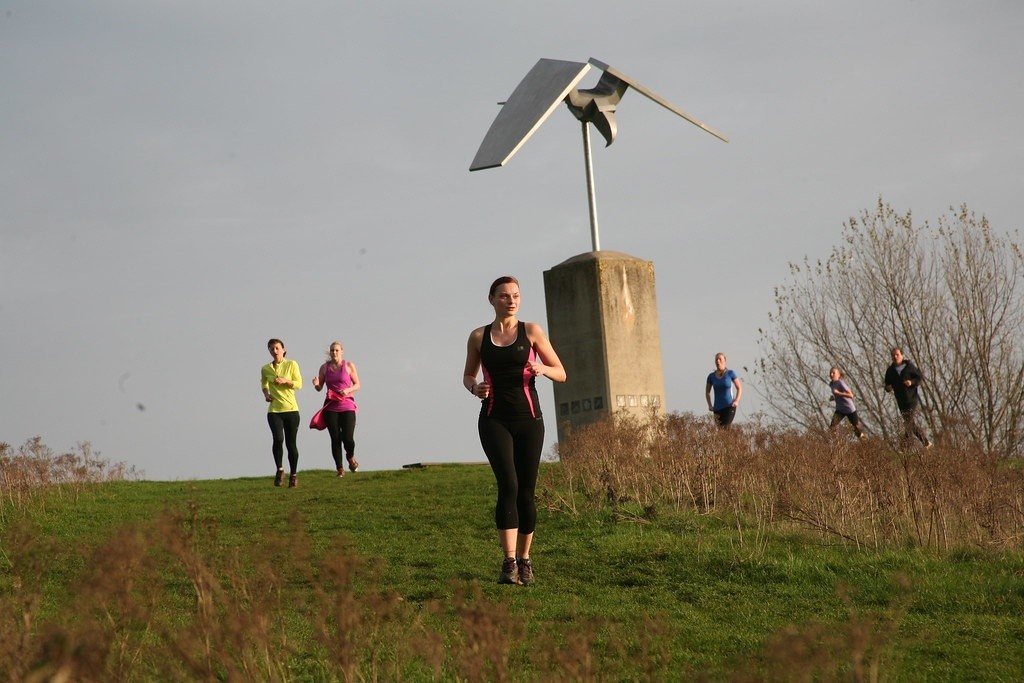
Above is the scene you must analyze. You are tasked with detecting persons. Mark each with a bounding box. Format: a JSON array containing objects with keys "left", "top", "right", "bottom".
[
  {"left": 312, "top": 341, "right": 360, "bottom": 477},
  {"left": 705, "top": 353, "right": 741, "bottom": 427},
  {"left": 827, "top": 366, "right": 863, "bottom": 441},
  {"left": 463, "top": 276, "right": 566, "bottom": 583},
  {"left": 884, "top": 346, "right": 933, "bottom": 451},
  {"left": 261, "top": 339, "right": 303, "bottom": 486}
]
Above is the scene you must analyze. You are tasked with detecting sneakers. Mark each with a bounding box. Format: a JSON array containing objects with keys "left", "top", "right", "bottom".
[
  {"left": 516, "top": 554, "right": 535, "bottom": 586},
  {"left": 496, "top": 557, "right": 519, "bottom": 585}
]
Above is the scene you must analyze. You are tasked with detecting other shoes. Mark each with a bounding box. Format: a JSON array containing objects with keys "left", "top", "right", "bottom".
[
  {"left": 347, "top": 456, "right": 358, "bottom": 472},
  {"left": 287, "top": 474, "right": 298, "bottom": 489},
  {"left": 923, "top": 442, "right": 932, "bottom": 451},
  {"left": 336, "top": 468, "right": 345, "bottom": 478},
  {"left": 274, "top": 469, "right": 285, "bottom": 487}
]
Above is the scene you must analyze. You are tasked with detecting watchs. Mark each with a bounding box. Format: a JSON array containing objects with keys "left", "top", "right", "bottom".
[{"left": 468, "top": 383, "right": 477, "bottom": 395}]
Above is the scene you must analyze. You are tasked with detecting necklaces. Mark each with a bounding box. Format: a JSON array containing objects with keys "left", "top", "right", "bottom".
[{"left": 714, "top": 369, "right": 725, "bottom": 376}]
